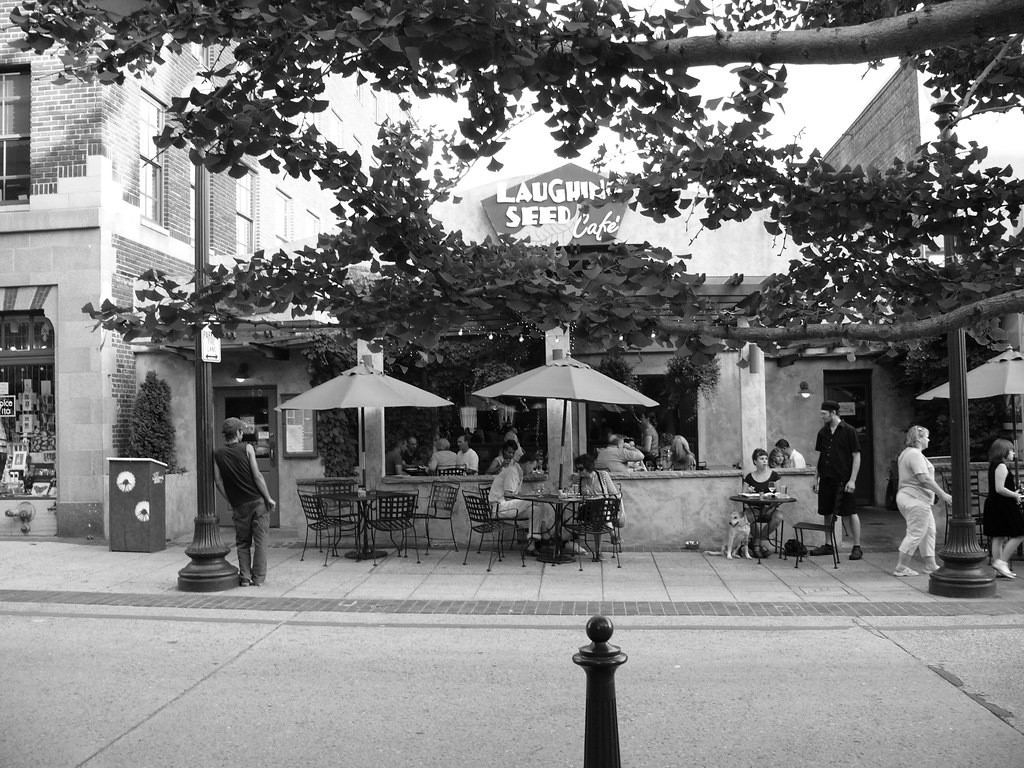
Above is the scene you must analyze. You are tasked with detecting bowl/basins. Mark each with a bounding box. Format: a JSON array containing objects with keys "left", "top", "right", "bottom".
[{"left": 685, "top": 540, "right": 701, "bottom": 549}]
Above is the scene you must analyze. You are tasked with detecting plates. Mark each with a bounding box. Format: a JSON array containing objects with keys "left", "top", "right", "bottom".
[{"left": 739, "top": 493, "right": 761, "bottom": 498}]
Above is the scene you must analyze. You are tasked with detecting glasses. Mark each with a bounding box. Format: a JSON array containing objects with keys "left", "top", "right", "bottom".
[
  {"left": 917, "top": 426, "right": 924, "bottom": 433},
  {"left": 576, "top": 467, "right": 586, "bottom": 472}
]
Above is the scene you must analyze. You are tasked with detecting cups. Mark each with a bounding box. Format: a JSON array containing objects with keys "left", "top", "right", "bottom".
[
  {"left": 369, "top": 476, "right": 377, "bottom": 495},
  {"left": 780, "top": 486, "right": 787, "bottom": 499}
]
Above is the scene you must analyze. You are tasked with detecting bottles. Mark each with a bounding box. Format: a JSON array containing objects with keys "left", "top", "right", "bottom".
[
  {"left": 358, "top": 485, "right": 366, "bottom": 497},
  {"left": 558, "top": 487, "right": 568, "bottom": 498}
]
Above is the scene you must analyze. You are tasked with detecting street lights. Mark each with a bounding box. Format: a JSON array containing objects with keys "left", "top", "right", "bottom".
[
  {"left": 917, "top": 48, "right": 997, "bottom": 601},
  {"left": 173, "top": 72, "right": 241, "bottom": 594}
]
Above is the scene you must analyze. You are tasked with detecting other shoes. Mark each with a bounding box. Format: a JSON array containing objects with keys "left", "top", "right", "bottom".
[
  {"left": 992, "top": 559, "right": 1016, "bottom": 580},
  {"left": 748, "top": 539, "right": 762, "bottom": 557},
  {"left": 241, "top": 579, "right": 249, "bottom": 587},
  {"left": 994, "top": 570, "right": 1016, "bottom": 577},
  {"left": 761, "top": 544, "right": 771, "bottom": 557},
  {"left": 595, "top": 553, "right": 606, "bottom": 560},
  {"left": 610, "top": 537, "right": 624, "bottom": 544},
  {"left": 254, "top": 582, "right": 262, "bottom": 586},
  {"left": 849, "top": 548, "right": 863, "bottom": 560},
  {"left": 809, "top": 544, "right": 834, "bottom": 556},
  {"left": 524, "top": 549, "right": 541, "bottom": 556}
]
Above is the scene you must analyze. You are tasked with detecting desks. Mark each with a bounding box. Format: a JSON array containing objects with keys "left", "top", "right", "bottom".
[
  {"left": 404, "top": 466, "right": 428, "bottom": 476},
  {"left": 727, "top": 493, "right": 797, "bottom": 563},
  {"left": 506, "top": 493, "right": 602, "bottom": 564},
  {"left": 312, "top": 490, "right": 412, "bottom": 561}
]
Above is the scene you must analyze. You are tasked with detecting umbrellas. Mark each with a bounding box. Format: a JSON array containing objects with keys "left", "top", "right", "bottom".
[
  {"left": 273, "top": 358, "right": 454, "bottom": 553},
  {"left": 915, "top": 343, "right": 1023, "bottom": 490}
]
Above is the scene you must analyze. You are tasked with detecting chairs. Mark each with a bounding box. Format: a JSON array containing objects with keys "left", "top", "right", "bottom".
[
  {"left": 435, "top": 463, "right": 467, "bottom": 476},
  {"left": 297, "top": 487, "right": 361, "bottom": 567},
  {"left": 568, "top": 482, "right": 622, "bottom": 559},
  {"left": 365, "top": 489, "right": 422, "bottom": 567},
  {"left": 315, "top": 477, "right": 361, "bottom": 556},
  {"left": 794, "top": 481, "right": 846, "bottom": 570},
  {"left": 559, "top": 490, "right": 625, "bottom": 573},
  {"left": 738, "top": 481, "right": 786, "bottom": 560},
  {"left": 477, "top": 483, "right": 529, "bottom": 559},
  {"left": 462, "top": 489, "right": 528, "bottom": 571},
  {"left": 403, "top": 480, "right": 461, "bottom": 556}
]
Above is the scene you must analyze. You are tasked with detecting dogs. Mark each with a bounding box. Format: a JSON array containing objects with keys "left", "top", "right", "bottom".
[{"left": 705, "top": 509, "right": 752, "bottom": 560}]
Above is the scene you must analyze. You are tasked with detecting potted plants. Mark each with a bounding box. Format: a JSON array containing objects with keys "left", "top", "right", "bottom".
[{"left": 126, "top": 370, "right": 189, "bottom": 540}]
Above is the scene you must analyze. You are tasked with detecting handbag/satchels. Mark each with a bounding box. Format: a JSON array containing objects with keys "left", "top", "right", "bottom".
[{"left": 577, "top": 504, "right": 595, "bottom": 521}]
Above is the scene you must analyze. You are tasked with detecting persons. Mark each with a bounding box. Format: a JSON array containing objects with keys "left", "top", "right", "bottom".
[
  {"left": 560, "top": 452, "right": 620, "bottom": 542},
  {"left": 894, "top": 425, "right": 952, "bottom": 576},
  {"left": 739, "top": 448, "right": 784, "bottom": 557},
  {"left": 487, "top": 454, "right": 557, "bottom": 557},
  {"left": 386, "top": 420, "right": 522, "bottom": 475},
  {"left": 768, "top": 449, "right": 786, "bottom": 468},
  {"left": 810, "top": 401, "right": 864, "bottom": 560},
  {"left": 982, "top": 440, "right": 1024, "bottom": 578},
  {"left": 592, "top": 407, "right": 697, "bottom": 471},
  {"left": 471, "top": 352, "right": 662, "bottom": 557},
  {"left": 212, "top": 416, "right": 276, "bottom": 586},
  {"left": 775, "top": 439, "right": 806, "bottom": 468}
]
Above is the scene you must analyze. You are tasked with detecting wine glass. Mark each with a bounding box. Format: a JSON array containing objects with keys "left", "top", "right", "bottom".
[
  {"left": 537, "top": 483, "right": 545, "bottom": 497},
  {"left": 768, "top": 481, "right": 776, "bottom": 493},
  {"left": 572, "top": 485, "right": 579, "bottom": 500}
]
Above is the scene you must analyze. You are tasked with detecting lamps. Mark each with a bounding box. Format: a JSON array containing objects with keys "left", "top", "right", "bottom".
[
  {"left": 797, "top": 382, "right": 814, "bottom": 398},
  {"left": 231, "top": 363, "right": 251, "bottom": 382}
]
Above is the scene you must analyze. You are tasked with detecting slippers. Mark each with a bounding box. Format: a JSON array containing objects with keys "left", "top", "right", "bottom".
[
  {"left": 894, "top": 567, "right": 918, "bottom": 576},
  {"left": 923, "top": 567, "right": 940, "bottom": 574}
]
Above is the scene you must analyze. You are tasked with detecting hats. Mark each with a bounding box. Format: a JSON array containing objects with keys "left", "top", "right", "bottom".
[
  {"left": 821, "top": 401, "right": 841, "bottom": 413},
  {"left": 221, "top": 417, "right": 247, "bottom": 434}
]
